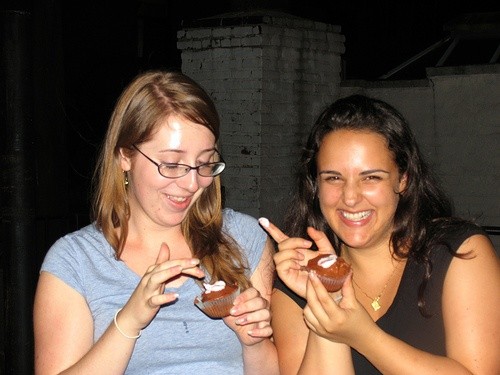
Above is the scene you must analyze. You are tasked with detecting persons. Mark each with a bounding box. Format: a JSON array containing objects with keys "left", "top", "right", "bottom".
[
  {"left": 33, "top": 69, "right": 280, "bottom": 375},
  {"left": 258, "top": 95, "right": 500, "bottom": 374}
]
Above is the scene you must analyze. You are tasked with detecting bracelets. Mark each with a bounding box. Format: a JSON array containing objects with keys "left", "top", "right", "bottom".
[{"left": 113, "top": 307, "right": 141, "bottom": 342}]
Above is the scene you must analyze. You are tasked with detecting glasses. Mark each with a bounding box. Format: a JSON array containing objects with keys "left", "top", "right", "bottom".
[{"left": 131, "top": 143, "right": 227, "bottom": 179}]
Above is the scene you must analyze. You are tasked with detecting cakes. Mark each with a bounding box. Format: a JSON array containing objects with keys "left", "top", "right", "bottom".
[
  {"left": 194, "top": 280, "right": 240, "bottom": 318},
  {"left": 307, "top": 254, "right": 352, "bottom": 291}
]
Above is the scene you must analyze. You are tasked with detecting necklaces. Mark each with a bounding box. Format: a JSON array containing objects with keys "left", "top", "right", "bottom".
[{"left": 340, "top": 242, "right": 404, "bottom": 312}]
